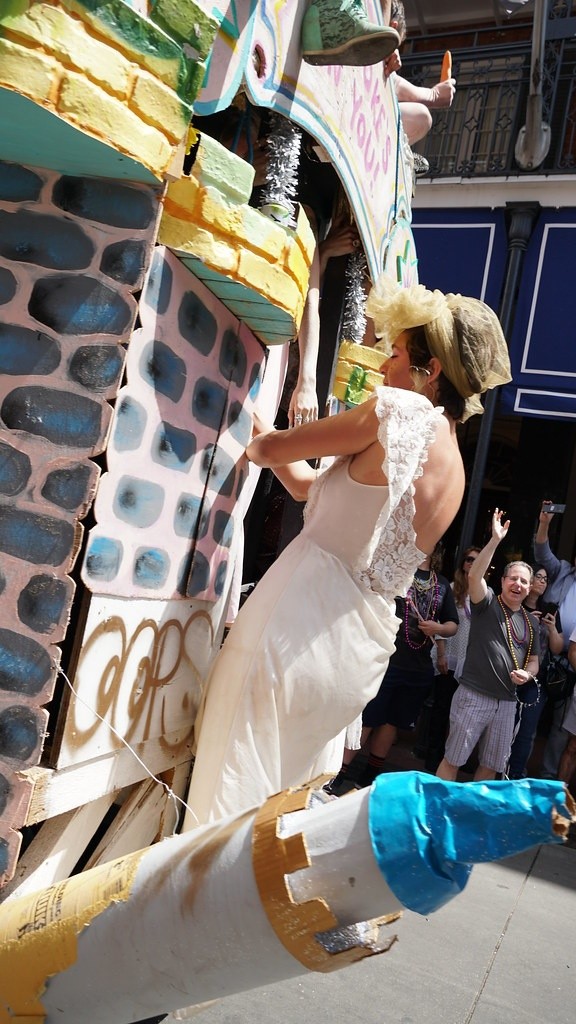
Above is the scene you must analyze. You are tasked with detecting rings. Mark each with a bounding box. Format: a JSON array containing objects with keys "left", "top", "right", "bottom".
[
  {"left": 294, "top": 417, "right": 302, "bottom": 422},
  {"left": 303, "top": 419, "right": 309, "bottom": 423},
  {"left": 352, "top": 239, "right": 359, "bottom": 247}
]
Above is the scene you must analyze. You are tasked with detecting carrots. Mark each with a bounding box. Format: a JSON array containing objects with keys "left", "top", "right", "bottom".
[{"left": 440, "top": 50, "right": 452, "bottom": 82}]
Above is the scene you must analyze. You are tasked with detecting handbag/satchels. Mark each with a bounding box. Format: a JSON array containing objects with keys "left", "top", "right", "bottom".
[{"left": 537, "top": 651, "right": 576, "bottom": 700}]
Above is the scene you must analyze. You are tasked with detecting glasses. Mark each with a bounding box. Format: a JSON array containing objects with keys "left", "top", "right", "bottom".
[
  {"left": 466, "top": 556, "right": 475, "bottom": 563},
  {"left": 535, "top": 574, "right": 548, "bottom": 583}
]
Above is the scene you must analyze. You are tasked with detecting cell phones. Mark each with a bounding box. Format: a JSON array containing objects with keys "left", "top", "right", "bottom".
[
  {"left": 543, "top": 503, "right": 566, "bottom": 513},
  {"left": 541, "top": 601, "right": 558, "bottom": 622}
]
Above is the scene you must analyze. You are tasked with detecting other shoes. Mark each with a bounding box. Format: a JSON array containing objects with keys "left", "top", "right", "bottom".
[
  {"left": 354, "top": 773, "right": 373, "bottom": 789},
  {"left": 299, "top": 0, "right": 399, "bottom": 67},
  {"left": 494, "top": 771, "right": 523, "bottom": 780},
  {"left": 324, "top": 772, "right": 344, "bottom": 791}
]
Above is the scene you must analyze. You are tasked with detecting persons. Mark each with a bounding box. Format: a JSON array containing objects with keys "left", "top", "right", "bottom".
[
  {"left": 332, "top": 543, "right": 459, "bottom": 789},
  {"left": 393, "top": 547, "right": 488, "bottom": 761},
  {"left": 183, "top": 286, "right": 511, "bottom": 832},
  {"left": 498, "top": 565, "right": 563, "bottom": 781},
  {"left": 219, "top": 95, "right": 362, "bottom": 429},
  {"left": 435, "top": 507, "right": 540, "bottom": 782},
  {"left": 537, "top": 500, "right": 576, "bottom": 837},
  {"left": 302, "top": 0, "right": 456, "bottom": 177}
]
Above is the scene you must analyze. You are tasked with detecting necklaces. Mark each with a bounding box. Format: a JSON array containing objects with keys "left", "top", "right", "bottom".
[
  {"left": 464, "top": 604, "right": 471, "bottom": 619},
  {"left": 497, "top": 594, "right": 533, "bottom": 671},
  {"left": 405, "top": 571, "right": 439, "bottom": 649}
]
